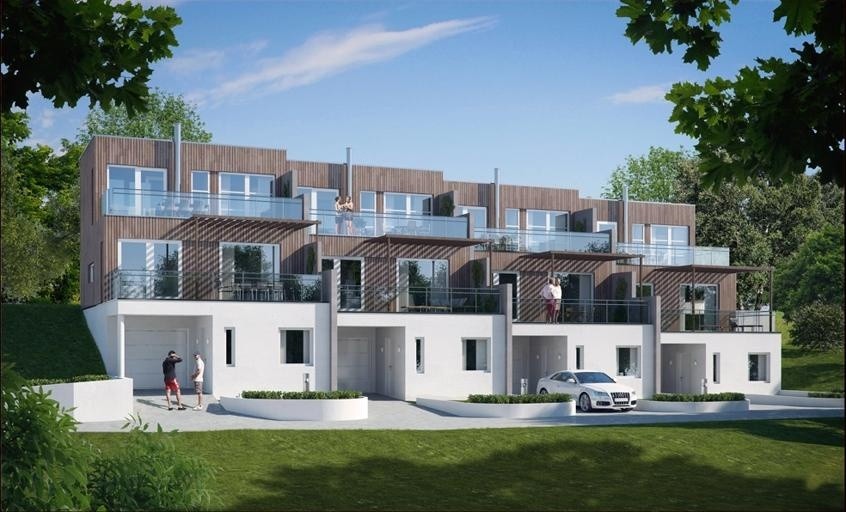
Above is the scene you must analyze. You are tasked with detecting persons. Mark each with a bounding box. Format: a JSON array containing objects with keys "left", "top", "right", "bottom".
[
  {"left": 190, "top": 351, "right": 205, "bottom": 411},
  {"left": 539, "top": 277, "right": 556, "bottom": 324},
  {"left": 334, "top": 196, "right": 343, "bottom": 236},
  {"left": 553, "top": 278, "right": 563, "bottom": 324},
  {"left": 340, "top": 196, "right": 354, "bottom": 236},
  {"left": 161, "top": 349, "right": 187, "bottom": 411}
]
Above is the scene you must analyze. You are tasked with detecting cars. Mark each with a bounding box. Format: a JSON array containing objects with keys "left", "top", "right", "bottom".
[{"left": 534, "top": 368, "right": 638, "bottom": 413}]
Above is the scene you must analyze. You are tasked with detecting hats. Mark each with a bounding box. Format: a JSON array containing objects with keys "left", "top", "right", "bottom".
[{"left": 193, "top": 351, "right": 200, "bottom": 355}]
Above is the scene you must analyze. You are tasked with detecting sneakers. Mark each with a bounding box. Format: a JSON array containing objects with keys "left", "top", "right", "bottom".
[
  {"left": 169, "top": 407, "right": 186, "bottom": 410},
  {"left": 192, "top": 404, "right": 202, "bottom": 411}
]
